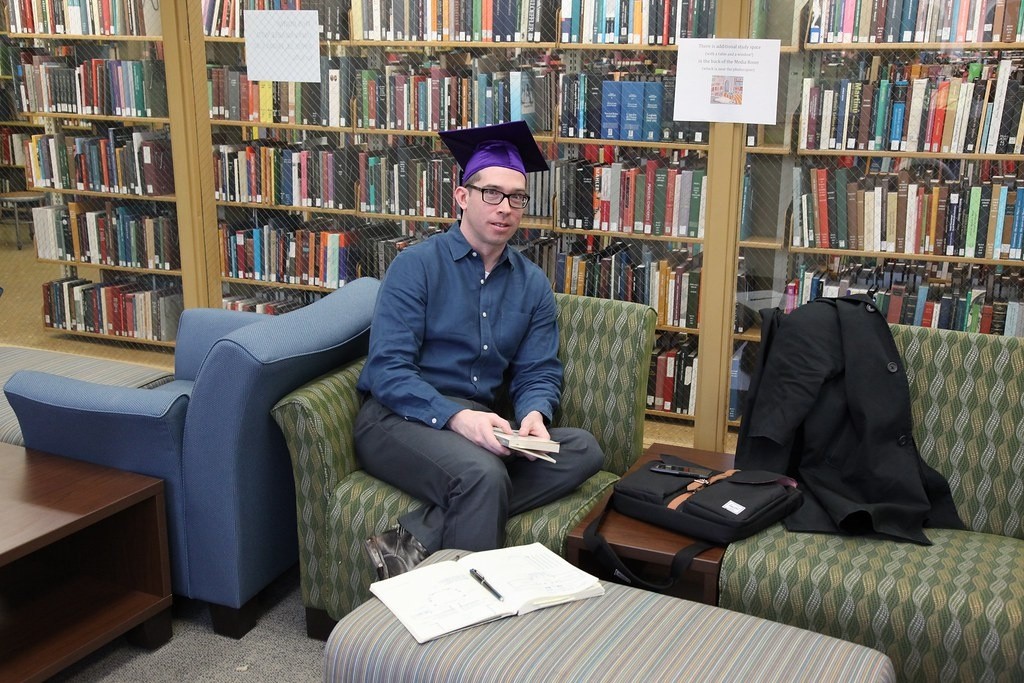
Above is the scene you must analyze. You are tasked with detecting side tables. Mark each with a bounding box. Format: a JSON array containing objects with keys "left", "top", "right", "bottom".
[
  {"left": 565, "top": 442, "right": 735, "bottom": 607},
  {"left": 0, "top": 441, "right": 174, "bottom": 683}
]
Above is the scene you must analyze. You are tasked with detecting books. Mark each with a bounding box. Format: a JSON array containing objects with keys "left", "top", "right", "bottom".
[
  {"left": 783, "top": 0, "right": 1023, "bottom": 339},
  {"left": 202, "top": 1, "right": 758, "bottom": 421},
  {"left": 367, "top": 541, "right": 606, "bottom": 645},
  {"left": 0, "top": 0, "right": 184, "bottom": 343},
  {"left": 491, "top": 426, "right": 561, "bottom": 465}
]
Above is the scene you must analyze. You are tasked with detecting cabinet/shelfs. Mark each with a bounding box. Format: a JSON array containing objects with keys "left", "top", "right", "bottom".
[
  {"left": 0, "top": 31, "right": 98, "bottom": 212},
  {"left": 4, "top": 0, "right": 1024, "bottom": 428}
]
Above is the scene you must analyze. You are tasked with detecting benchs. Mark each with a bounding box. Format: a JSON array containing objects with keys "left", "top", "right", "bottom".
[{"left": 323, "top": 549, "right": 896, "bottom": 683}]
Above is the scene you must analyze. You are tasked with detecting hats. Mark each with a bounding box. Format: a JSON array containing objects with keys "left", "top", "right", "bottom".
[{"left": 438, "top": 120, "right": 550, "bottom": 184}]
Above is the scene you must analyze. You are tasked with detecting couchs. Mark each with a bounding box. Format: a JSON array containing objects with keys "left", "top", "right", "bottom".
[
  {"left": 716, "top": 323, "right": 1024, "bottom": 683},
  {"left": 270, "top": 292, "right": 658, "bottom": 641},
  {"left": 3, "top": 277, "right": 381, "bottom": 641},
  {"left": 0, "top": 345, "right": 175, "bottom": 446}
]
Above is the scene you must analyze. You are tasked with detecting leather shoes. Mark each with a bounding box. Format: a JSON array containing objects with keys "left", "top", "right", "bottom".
[{"left": 365, "top": 528, "right": 428, "bottom": 578}]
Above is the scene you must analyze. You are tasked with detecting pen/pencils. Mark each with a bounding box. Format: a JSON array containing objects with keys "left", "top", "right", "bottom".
[{"left": 471, "top": 568, "right": 503, "bottom": 599}]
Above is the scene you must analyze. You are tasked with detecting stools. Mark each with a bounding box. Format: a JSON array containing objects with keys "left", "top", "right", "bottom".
[{"left": 0, "top": 191, "right": 47, "bottom": 250}]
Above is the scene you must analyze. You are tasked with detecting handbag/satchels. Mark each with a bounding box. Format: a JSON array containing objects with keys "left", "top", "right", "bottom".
[{"left": 585, "top": 454, "right": 803, "bottom": 592}]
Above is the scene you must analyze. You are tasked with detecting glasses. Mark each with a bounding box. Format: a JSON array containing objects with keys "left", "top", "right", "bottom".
[{"left": 464, "top": 184, "right": 530, "bottom": 208}]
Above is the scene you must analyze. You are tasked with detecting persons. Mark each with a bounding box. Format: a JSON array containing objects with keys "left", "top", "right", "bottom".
[{"left": 352, "top": 141, "right": 604, "bottom": 581}]
